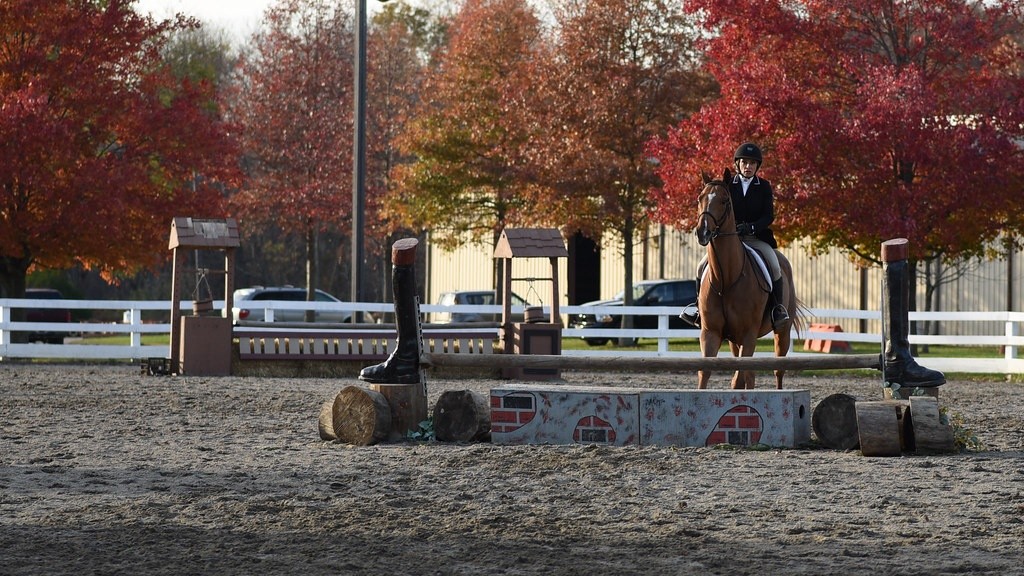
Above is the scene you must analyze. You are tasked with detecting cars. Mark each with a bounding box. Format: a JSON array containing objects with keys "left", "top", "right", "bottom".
[{"left": 26, "top": 289, "right": 69, "bottom": 345}]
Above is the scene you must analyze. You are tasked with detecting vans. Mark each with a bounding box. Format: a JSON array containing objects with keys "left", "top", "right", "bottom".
[{"left": 220, "top": 285, "right": 375, "bottom": 324}]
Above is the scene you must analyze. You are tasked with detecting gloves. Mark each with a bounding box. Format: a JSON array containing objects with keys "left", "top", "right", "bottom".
[{"left": 737, "top": 221, "right": 754, "bottom": 236}]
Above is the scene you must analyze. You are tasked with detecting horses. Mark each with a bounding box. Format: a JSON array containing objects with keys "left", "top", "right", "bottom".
[{"left": 696, "top": 166, "right": 797, "bottom": 391}]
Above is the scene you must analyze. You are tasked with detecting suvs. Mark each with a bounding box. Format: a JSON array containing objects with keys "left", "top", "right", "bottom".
[
  {"left": 569, "top": 280, "right": 701, "bottom": 346},
  {"left": 434, "top": 290, "right": 532, "bottom": 323}
]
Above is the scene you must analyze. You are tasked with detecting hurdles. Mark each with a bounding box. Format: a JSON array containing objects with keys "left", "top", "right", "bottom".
[{"left": 319, "top": 237, "right": 954, "bottom": 456}]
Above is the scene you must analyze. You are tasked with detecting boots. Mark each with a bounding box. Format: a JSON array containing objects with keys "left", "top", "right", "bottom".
[
  {"left": 772, "top": 276, "right": 790, "bottom": 334},
  {"left": 882, "top": 259, "right": 947, "bottom": 387},
  {"left": 358, "top": 265, "right": 431, "bottom": 384}
]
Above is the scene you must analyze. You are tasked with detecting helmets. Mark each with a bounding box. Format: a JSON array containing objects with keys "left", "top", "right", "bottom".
[{"left": 734, "top": 143, "right": 762, "bottom": 162}]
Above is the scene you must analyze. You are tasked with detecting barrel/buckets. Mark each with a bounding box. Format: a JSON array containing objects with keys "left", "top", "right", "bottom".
[
  {"left": 523, "top": 286, "right": 544, "bottom": 323},
  {"left": 192, "top": 275, "right": 214, "bottom": 316}
]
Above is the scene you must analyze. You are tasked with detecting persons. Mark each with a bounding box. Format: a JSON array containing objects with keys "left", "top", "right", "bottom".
[{"left": 679, "top": 142, "right": 790, "bottom": 329}]
